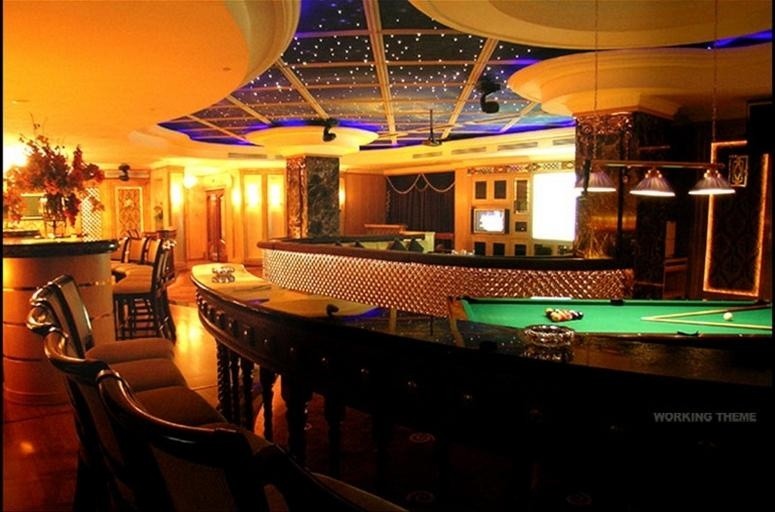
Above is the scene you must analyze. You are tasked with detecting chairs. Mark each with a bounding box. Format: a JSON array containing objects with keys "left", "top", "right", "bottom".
[{"left": 29, "top": 229, "right": 433, "bottom": 512}]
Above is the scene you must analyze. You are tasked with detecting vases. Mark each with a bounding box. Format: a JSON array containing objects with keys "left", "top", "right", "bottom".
[{"left": 44, "top": 205, "right": 83, "bottom": 238}]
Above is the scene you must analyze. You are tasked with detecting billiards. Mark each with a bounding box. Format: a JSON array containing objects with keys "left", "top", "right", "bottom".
[
  {"left": 545, "top": 308, "right": 585, "bottom": 323},
  {"left": 724, "top": 312, "right": 734, "bottom": 321}
]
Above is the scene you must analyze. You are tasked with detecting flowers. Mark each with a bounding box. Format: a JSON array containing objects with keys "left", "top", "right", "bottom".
[{"left": 3, "top": 114, "right": 105, "bottom": 228}]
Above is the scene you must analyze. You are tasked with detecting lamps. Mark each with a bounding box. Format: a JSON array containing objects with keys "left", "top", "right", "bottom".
[
  {"left": 323, "top": 116, "right": 340, "bottom": 141},
  {"left": 575, "top": 0, "right": 736, "bottom": 196},
  {"left": 480, "top": 84, "right": 501, "bottom": 112}
]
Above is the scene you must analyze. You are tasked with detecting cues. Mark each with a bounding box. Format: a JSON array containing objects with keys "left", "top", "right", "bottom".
[
  {"left": 640, "top": 304, "right": 772, "bottom": 319},
  {"left": 641, "top": 318, "right": 772, "bottom": 331}
]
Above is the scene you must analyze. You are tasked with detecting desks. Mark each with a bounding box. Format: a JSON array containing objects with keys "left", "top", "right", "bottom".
[{"left": 445, "top": 296, "right": 773, "bottom": 334}]
